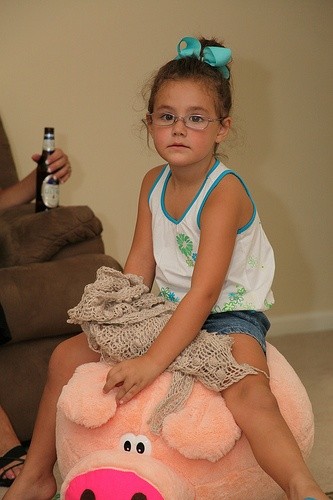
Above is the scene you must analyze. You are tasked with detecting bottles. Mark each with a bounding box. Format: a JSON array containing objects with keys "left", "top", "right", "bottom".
[{"left": 35, "top": 127, "right": 60, "bottom": 213}]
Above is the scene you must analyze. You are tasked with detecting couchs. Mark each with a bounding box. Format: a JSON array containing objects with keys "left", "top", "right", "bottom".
[{"left": 0, "top": 116, "right": 123, "bottom": 448}]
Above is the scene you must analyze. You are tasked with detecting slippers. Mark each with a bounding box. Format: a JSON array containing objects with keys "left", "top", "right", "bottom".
[{"left": 0, "top": 445, "right": 28, "bottom": 488}]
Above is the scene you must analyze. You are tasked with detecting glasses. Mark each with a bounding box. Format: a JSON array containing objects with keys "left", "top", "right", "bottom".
[{"left": 148, "top": 111, "right": 224, "bottom": 130}]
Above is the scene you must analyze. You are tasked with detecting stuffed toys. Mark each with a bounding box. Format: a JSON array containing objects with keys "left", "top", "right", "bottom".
[{"left": 54, "top": 333, "right": 316, "bottom": 500}]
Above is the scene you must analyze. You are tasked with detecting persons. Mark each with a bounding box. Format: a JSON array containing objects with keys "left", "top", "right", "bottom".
[
  {"left": 1, "top": 34, "right": 333, "bottom": 500},
  {"left": 0, "top": 149, "right": 72, "bottom": 486}
]
